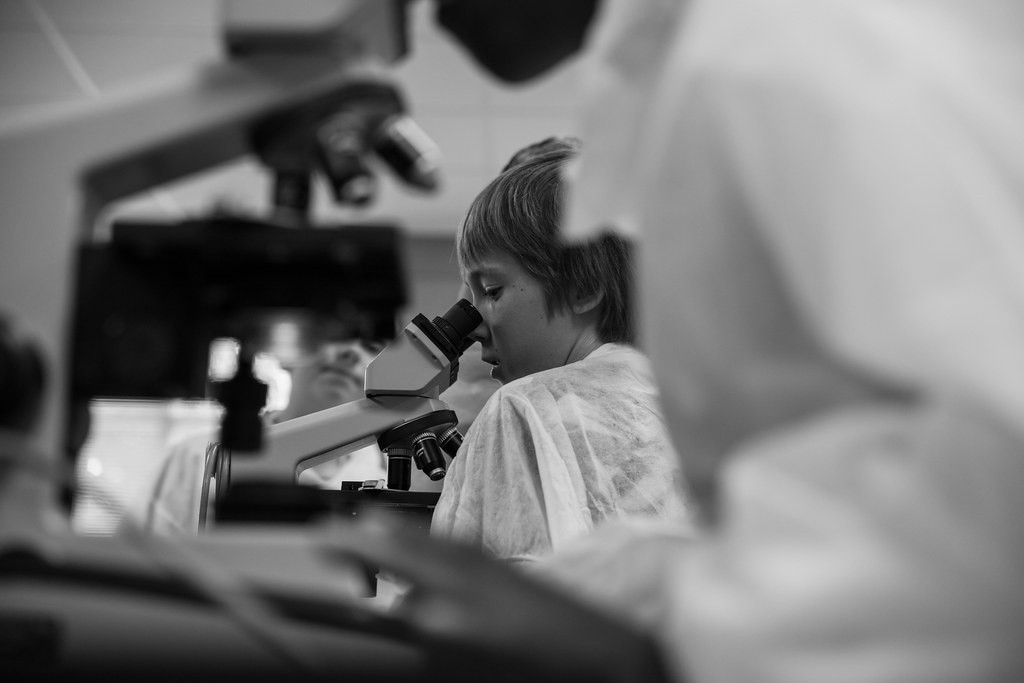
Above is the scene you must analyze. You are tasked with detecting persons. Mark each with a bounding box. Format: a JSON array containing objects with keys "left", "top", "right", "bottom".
[
  {"left": 118, "top": 340, "right": 388, "bottom": 536},
  {"left": 525, "top": 0, "right": 1024, "bottom": 683},
  {"left": 428, "top": 139, "right": 685, "bottom": 572}
]
